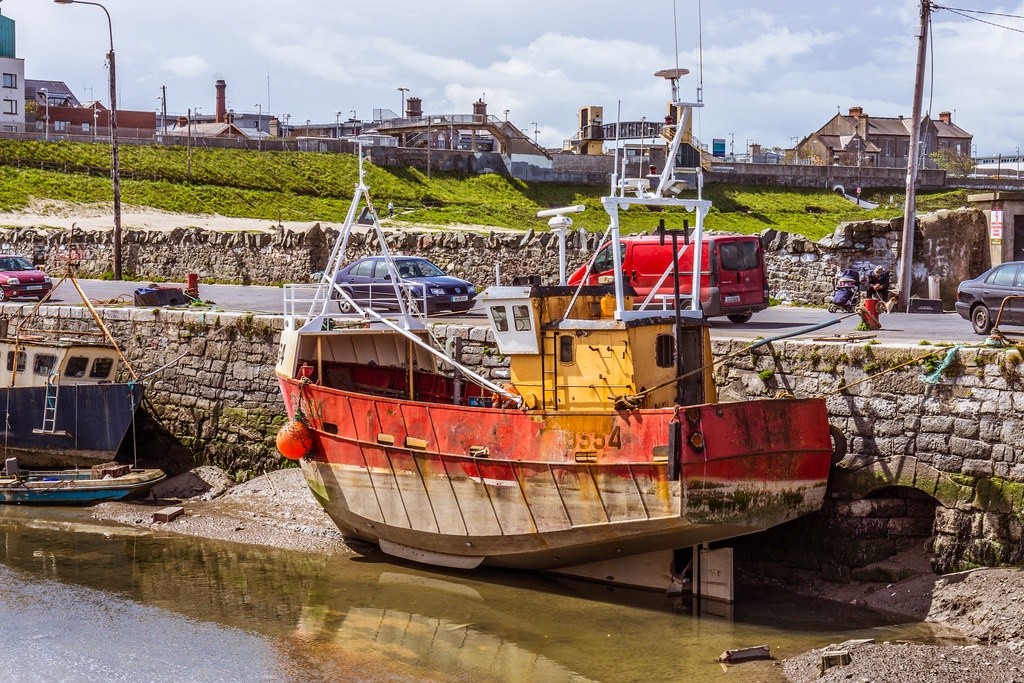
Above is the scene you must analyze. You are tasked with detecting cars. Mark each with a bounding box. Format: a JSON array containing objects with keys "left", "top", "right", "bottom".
[
  {"left": 0, "top": 255, "right": 53, "bottom": 302},
  {"left": 328, "top": 257, "right": 478, "bottom": 317},
  {"left": 955, "top": 261, "right": 1024, "bottom": 335},
  {"left": 721, "top": 157, "right": 736, "bottom": 162}
]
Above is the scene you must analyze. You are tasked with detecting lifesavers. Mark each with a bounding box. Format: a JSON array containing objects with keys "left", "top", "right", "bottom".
[{"left": 828, "top": 419, "right": 846, "bottom": 463}]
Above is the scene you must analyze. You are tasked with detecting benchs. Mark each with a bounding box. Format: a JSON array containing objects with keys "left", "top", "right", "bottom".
[{"left": 910, "top": 299, "right": 943, "bottom": 313}]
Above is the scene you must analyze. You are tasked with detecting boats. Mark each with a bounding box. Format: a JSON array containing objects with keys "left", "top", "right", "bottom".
[
  {"left": 0, "top": 315, "right": 146, "bottom": 466},
  {"left": 274, "top": 2, "right": 832, "bottom": 607},
  {"left": 0, "top": 457, "right": 167, "bottom": 502}
]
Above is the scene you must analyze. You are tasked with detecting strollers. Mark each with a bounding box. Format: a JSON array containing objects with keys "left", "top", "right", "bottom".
[{"left": 828, "top": 268, "right": 862, "bottom": 313}]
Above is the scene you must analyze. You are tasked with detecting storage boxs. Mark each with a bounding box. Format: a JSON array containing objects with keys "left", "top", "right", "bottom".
[
  {"left": 720, "top": 644, "right": 769, "bottom": 662},
  {"left": 821, "top": 650, "right": 848, "bottom": 669}
]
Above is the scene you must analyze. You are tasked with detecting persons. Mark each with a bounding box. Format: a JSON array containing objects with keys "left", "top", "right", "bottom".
[{"left": 866, "top": 264, "right": 890, "bottom": 314}]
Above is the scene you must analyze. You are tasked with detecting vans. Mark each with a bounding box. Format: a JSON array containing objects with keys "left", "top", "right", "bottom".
[{"left": 568, "top": 235, "right": 769, "bottom": 323}]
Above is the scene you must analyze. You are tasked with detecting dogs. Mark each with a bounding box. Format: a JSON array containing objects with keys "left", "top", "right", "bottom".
[{"left": 872, "top": 296, "right": 899, "bottom": 315}]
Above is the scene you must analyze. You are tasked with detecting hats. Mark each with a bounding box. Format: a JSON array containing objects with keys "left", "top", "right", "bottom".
[{"left": 873, "top": 265, "right": 886, "bottom": 276}]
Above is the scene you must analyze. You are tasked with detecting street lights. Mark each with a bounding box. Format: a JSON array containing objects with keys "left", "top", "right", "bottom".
[
  {"left": 792, "top": 136, "right": 798, "bottom": 165},
  {"left": 531, "top": 122, "right": 537, "bottom": 143},
  {"left": 195, "top": 106, "right": 202, "bottom": 130},
  {"left": 728, "top": 132, "right": 752, "bottom": 158},
  {"left": 54, "top": 0, "right": 122, "bottom": 279},
  {"left": 156, "top": 97, "right": 163, "bottom": 134},
  {"left": 1015, "top": 146, "right": 1019, "bottom": 179},
  {"left": 397, "top": 88, "right": 410, "bottom": 118},
  {"left": 286, "top": 113, "right": 291, "bottom": 136},
  {"left": 427, "top": 116, "right": 437, "bottom": 177},
  {"left": 226, "top": 113, "right": 231, "bottom": 138},
  {"left": 853, "top": 138, "right": 860, "bottom": 166},
  {"left": 41, "top": 88, "right": 48, "bottom": 140},
  {"left": 254, "top": 104, "right": 261, "bottom": 140},
  {"left": 971, "top": 144, "right": 977, "bottom": 163},
  {"left": 349, "top": 110, "right": 356, "bottom": 139}
]
